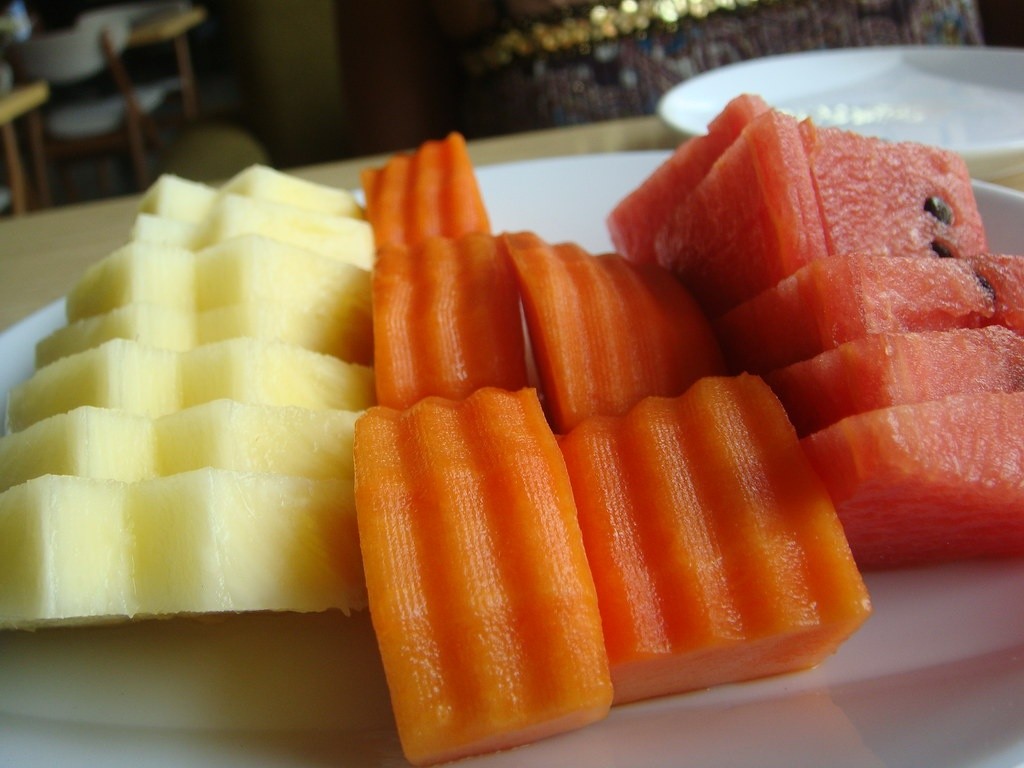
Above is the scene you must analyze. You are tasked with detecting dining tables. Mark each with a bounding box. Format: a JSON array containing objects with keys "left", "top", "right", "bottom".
[
  {"left": 3, "top": 81, "right": 47, "bottom": 213},
  {"left": 126, "top": 8, "right": 202, "bottom": 148},
  {"left": 1, "top": 117, "right": 1024, "bottom": 767}
]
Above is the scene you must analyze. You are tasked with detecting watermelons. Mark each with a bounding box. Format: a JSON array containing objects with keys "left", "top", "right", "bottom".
[{"left": 606, "top": 93, "right": 1024, "bottom": 572}]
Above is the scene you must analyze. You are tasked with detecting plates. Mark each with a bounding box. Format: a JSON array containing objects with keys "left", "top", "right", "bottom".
[
  {"left": 0, "top": 144, "right": 1020, "bottom": 768},
  {"left": 656, "top": 44, "right": 1024, "bottom": 180}
]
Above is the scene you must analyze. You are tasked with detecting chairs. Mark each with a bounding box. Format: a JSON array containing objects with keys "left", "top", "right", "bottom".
[{"left": 6, "top": 15, "right": 168, "bottom": 187}]
